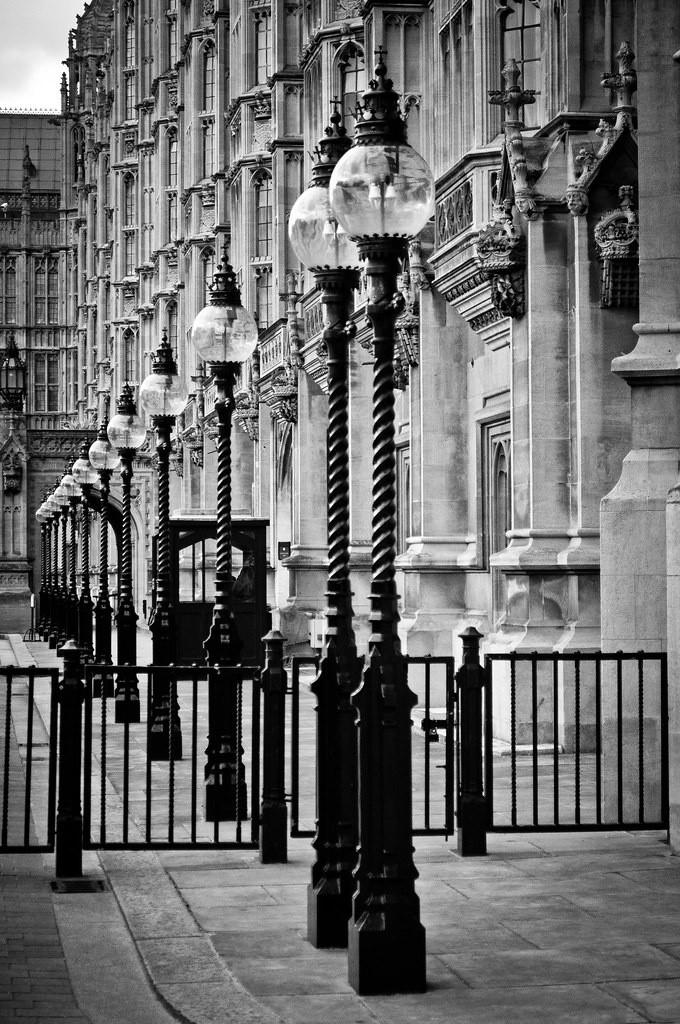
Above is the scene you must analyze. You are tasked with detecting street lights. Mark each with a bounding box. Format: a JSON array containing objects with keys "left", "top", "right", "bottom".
[
  {"left": 32, "top": 405, "right": 118, "bottom": 698},
  {"left": 325, "top": 38, "right": 439, "bottom": 996},
  {"left": 188, "top": 238, "right": 259, "bottom": 824},
  {"left": 286, "top": 95, "right": 363, "bottom": 952},
  {"left": 105, "top": 385, "right": 143, "bottom": 725},
  {"left": 137, "top": 327, "right": 190, "bottom": 761}
]
